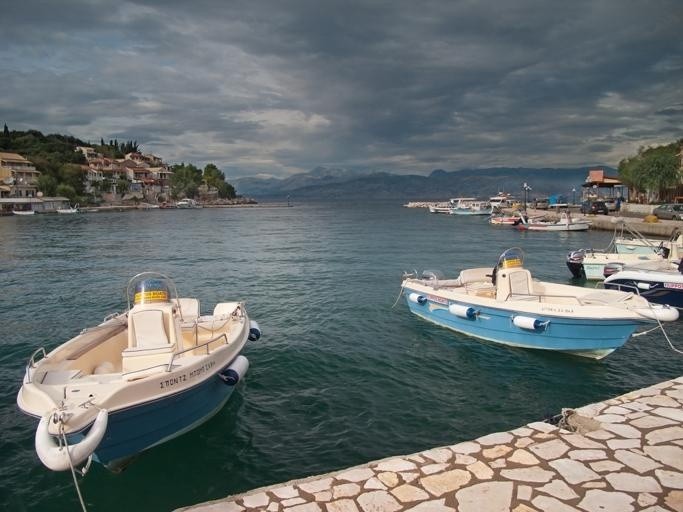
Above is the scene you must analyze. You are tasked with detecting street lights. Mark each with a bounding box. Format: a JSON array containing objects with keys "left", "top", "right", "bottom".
[
  {"left": 522, "top": 182, "right": 531, "bottom": 212},
  {"left": 572, "top": 184, "right": 576, "bottom": 205}
]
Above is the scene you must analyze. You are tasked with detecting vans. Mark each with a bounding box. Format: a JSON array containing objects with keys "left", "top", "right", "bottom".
[{"left": 488, "top": 197, "right": 507, "bottom": 206}]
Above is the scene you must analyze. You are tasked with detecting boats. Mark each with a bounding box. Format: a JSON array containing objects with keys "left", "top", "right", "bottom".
[
  {"left": 177, "top": 198, "right": 204, "bottom": 209},
  {"left": 566, "top": 249, "right": 679, "bottom": 283},
  {"left": 159, "top": 202, "right": 177, "bottom": 209},
  {"left": 13, "top": 269, "right": 260, "bottom": 471},
  {"left": 55, "top": 208, "right": 80, "bottom": 214},
  {"left": 10, "top": 208, "right": 39, "bottom": 215},
  {"left": 614, "top": 236, "right": 683, "bottom": 266},
  {"left": 427, "top": 201, "right": 591, "bottom": 232},
  {"left": 398, "top": 247, "right": 679, "bottom": 360},
  {"left": 603, "top": 264, "right": 682, "bottom": 302}
]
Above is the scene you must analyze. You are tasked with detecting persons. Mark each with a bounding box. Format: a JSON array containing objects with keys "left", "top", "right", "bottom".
[
  {"left": 615, "top": 198, "right": 622, "bottom": 217},
  {"left": 523, "top": 212, "right": 529, "bottom": 223}
]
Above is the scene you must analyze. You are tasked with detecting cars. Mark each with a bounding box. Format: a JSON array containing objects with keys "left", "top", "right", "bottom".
[
  {"left": 652, "top": 204, "right": 683, "bottom": 222},
  {"left": 580, "top": 200, "right": 607, "bottom": 215}
]
